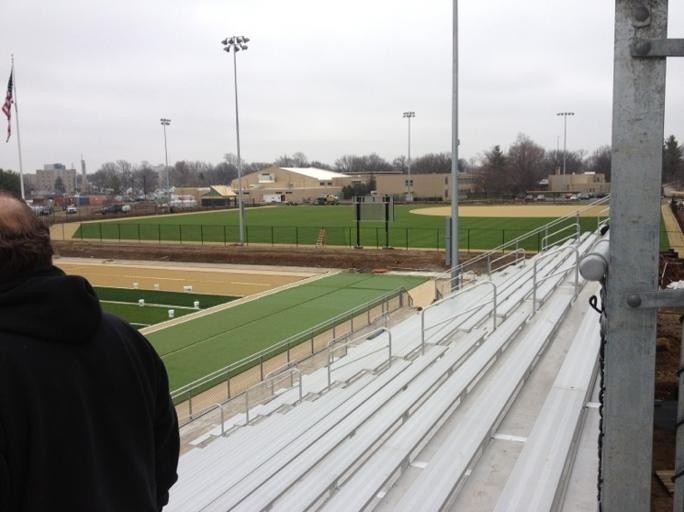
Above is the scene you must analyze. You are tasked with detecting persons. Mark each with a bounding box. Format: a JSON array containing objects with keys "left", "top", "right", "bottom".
[{"left": 0, "top": 191, "right": 181, "bottom": 512}]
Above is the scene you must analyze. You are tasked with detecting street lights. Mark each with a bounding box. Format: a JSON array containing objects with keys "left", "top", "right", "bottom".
[
  {"left": 160, "top": 118, "right": 171, "bottom": 214},
  {"left": 556, "top": 112, "right": 574, "bottom": 192},
  {"left": 222, "top": 36, "right": 250, "bottom": 246},
  {"left": 402, "top": 112, "right": 415, "bottom": 202}
]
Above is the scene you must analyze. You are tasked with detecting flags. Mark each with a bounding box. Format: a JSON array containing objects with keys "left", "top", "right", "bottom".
[{"left": 1, "top": 67, "right": 14, "bottom": 143}]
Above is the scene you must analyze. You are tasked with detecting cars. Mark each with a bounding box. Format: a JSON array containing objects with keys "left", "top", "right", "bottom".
[
  {"left": 565, "top": 192, "right": 608, "bottom": 200},
  {"left": 525, "top": 194, "right": 545, "bottom": 202},
  {"left": 39, "top": 204, "right": 76, "bottom": 216},
  {"left": 95, "top": 202, "right": 149, "bottom": 216}
]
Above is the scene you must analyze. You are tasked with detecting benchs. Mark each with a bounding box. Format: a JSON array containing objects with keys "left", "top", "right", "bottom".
[{"left": 161, "top": 228, "right": 611, "bottom": 512}]
